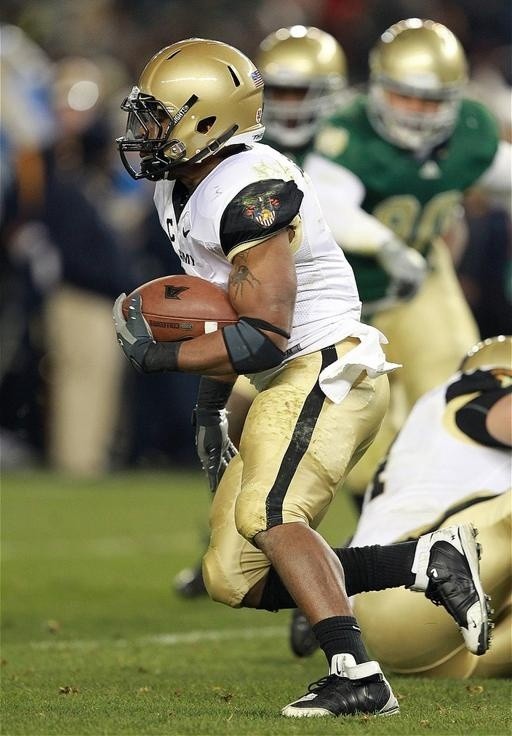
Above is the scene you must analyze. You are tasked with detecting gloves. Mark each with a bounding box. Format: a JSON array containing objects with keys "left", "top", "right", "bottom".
[
  {"left": 111, "top": 292, "right": 184, "bottom": 376},
  {"left": 193, "top": 376, "right": 237, "bottom": 493},
  {"left": 377, "top": 237, "right": 427, "bottom": 305}
]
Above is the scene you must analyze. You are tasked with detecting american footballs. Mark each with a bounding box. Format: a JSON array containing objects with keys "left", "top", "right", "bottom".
[{"left": 122, "top": 274, "right": 240, "bottom": 342}]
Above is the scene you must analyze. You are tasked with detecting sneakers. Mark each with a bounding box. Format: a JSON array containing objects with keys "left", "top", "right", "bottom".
[
  {"left": 404, "top": 521, "right": 495, "bottom": 655},
  {"left": 280, "top": 649, "right": 402, "bottom": 722}
]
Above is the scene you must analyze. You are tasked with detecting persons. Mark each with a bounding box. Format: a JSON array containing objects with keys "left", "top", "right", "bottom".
[
  {"left": 109, "top": 35, "right": 495, "bottom": 723},
  {"left": 341, "top": 332, "right": 511, "bottom": 679},
  {"left": 1, "top": 1, "right": 511, "bottom": 600}
]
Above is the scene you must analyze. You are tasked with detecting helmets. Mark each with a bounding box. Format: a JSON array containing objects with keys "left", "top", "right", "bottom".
[
  {"left": 364, "top": 17, "right": 471, "bottom": 152},
  {"left": 253, "top": 25, "right": 350, "bottom": 148},
  {"left": 115, "top": 37, "right": 265, "bottom": 181},
  {"left": 458, "top": 335, "right": 512, "bottom": 373}
]
